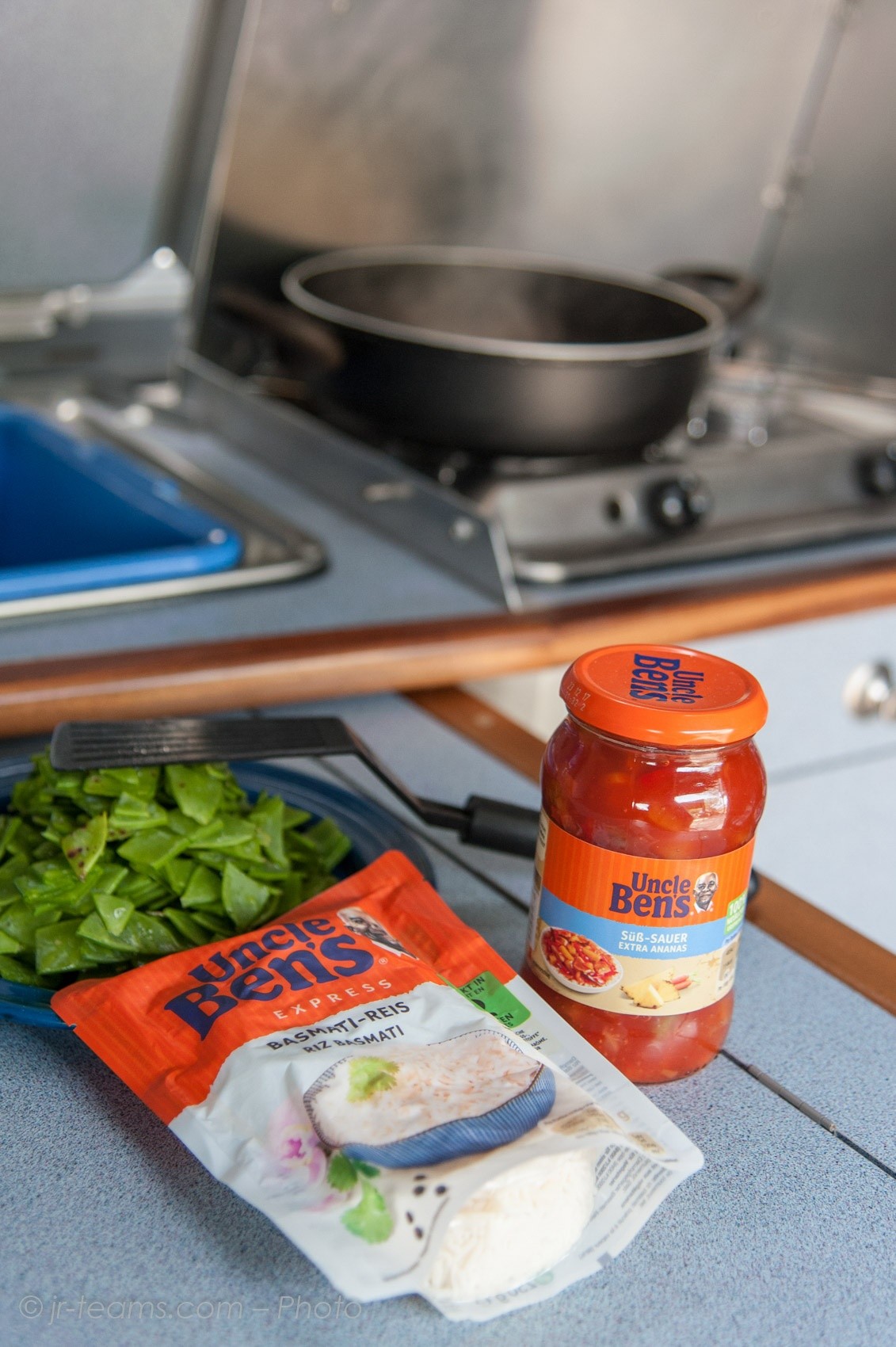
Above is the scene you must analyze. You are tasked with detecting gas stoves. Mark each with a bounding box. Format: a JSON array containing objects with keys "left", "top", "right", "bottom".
[{"left": 242, "top": 318, "right": 893, "bottom": 591}]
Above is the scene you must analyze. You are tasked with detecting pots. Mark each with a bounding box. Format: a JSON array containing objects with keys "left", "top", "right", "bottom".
[{"left": 286, "top": 239, "right": 762, "bottom": 460}]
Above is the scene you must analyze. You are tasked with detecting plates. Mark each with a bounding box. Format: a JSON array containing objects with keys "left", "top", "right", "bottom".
[{"left": 3, "top": 758, "right": 439, "bottom": 1030}]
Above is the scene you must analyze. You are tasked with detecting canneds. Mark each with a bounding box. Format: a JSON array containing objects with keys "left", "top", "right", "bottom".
[{"left": 522, "top": 635, "right": 779, "bottom": 1086}]
